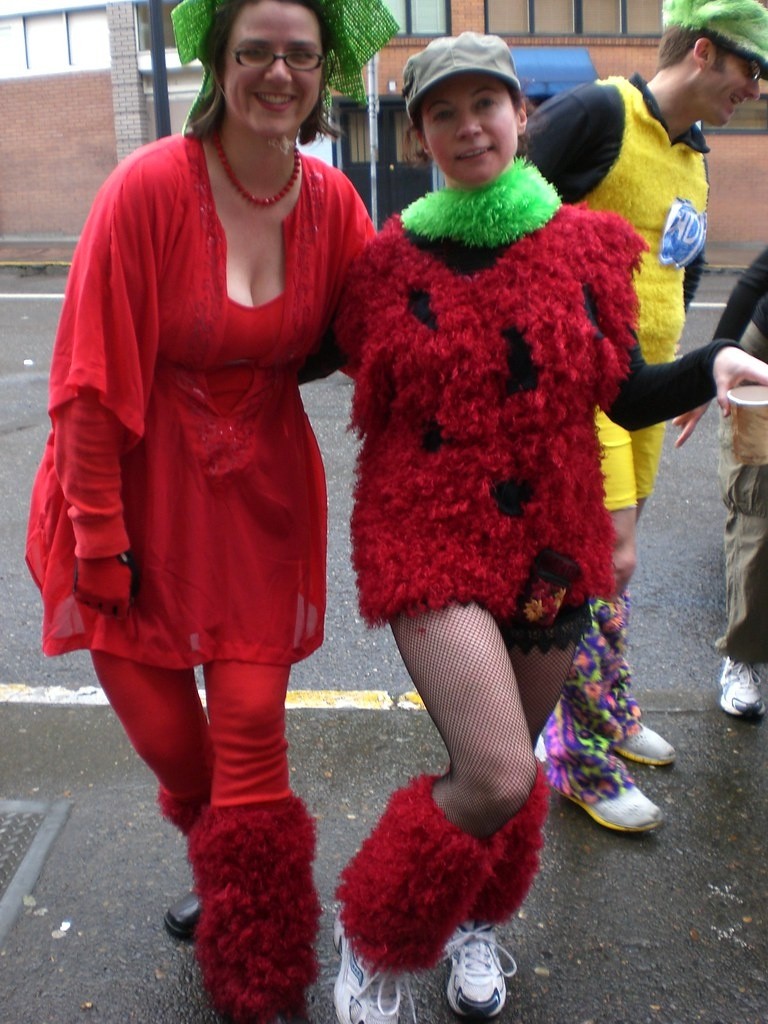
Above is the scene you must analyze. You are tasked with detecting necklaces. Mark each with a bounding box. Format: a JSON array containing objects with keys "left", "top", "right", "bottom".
[{"left": 212, "top": 128, "right": 300, "bottom": 208}]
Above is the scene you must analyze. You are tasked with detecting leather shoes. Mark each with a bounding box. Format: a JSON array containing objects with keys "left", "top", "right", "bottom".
[
  {"left": 276, "top": 1011, "right": 310, "bottom": 1024},
  {"left": 164, "top": 890, "right": 201, "bottom": 934}
]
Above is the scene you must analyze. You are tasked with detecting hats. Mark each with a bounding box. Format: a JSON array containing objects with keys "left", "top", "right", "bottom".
[
  {"left": 663, "top": 0, "right": 768, "bottom": 80},
  {"left": 402, "top": 31, "right": 521, "bottom": 128}
]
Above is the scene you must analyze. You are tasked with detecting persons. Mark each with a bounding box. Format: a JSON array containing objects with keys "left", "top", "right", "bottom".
[
  {"left": 515, "top": 0, "right": 768, "bottom": 833},
  {"left": 25, "top": 0, "right": 401, "bottom": 1024},
  {"left": 672, "top": 244, "right": 768, "bottom": 717},
  {"left": 296, "top": 32, "right": 768, "bottom": 1024}
]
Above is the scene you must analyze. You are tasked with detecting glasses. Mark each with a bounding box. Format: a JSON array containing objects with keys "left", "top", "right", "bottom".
[
  {"left": 227, "top": 46, "right": 323, "bottom": 72},
  {"left": 688, "top": 40, "right": 761, "bottom": 81}
]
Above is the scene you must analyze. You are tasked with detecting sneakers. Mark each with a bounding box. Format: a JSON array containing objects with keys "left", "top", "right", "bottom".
[
  {"left": 554, "top": 783, "right": 663, "bottom": 831},
  {"left": 333, "top": 905, "right": 424, "bottom": 1024},
  {"left": 719, "top": 656, "right": 766, "bottom": 715},
  {"left": 615, "top": 723, "right": 676, "bottom": 765},
  {"left": 435, "top": 919, "right": 517, "bottom": 1018}
]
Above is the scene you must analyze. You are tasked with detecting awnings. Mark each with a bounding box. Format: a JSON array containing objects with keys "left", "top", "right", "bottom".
[{"left": 510, "top": 46, "right": 602, "bottom": 99}]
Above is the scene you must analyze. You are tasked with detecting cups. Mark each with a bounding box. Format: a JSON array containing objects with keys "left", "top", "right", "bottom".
[{"left": 727, "top": 385, "right": 768, "bottom": 465}]
